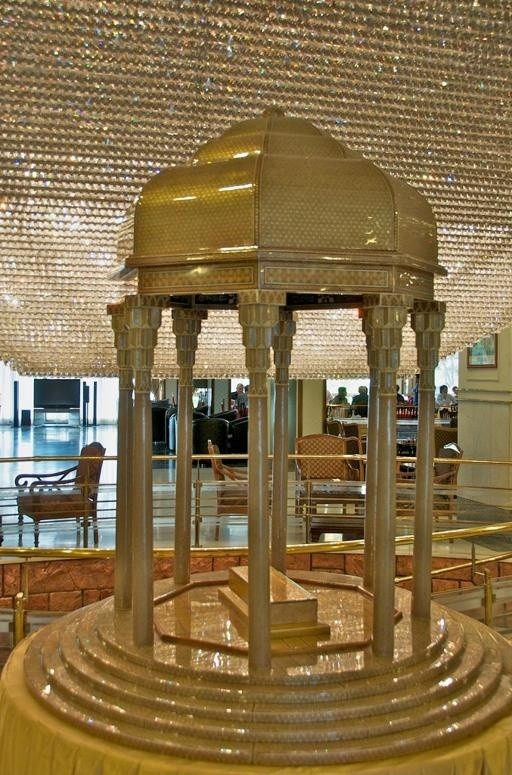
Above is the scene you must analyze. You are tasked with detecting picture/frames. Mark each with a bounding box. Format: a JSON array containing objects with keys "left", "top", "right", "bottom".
[{"left": 465, "top": 334, "right": 499, "bottom": 369}]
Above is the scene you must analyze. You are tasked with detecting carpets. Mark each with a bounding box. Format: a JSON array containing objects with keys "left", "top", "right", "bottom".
[{"left": 452, "top": 494, "right": 512, "bottom": 553}]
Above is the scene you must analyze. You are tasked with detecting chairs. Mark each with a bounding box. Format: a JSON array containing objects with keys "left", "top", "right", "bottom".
[
  {"left": 204, "top": 438, "right": 272, "bottom": 541},
  {"left": 398, "top": 441, "right": 464, "bottom": 485},
  {"left": 321, "top": 398, "right": 464, "bottom": 458},
  {"left": 294, "top": 432, "right": 365, "bottom": 519},
  {"left": 15, "top": 440, "right": 106, "bottom": 548},
  {"left": 301, "top": 480, "right": 366, "bottom": 542}
]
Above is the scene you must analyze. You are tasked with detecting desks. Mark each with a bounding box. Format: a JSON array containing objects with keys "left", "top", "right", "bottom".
[{"left": 297, "top": 477, "right": 462, "bottom": 520}]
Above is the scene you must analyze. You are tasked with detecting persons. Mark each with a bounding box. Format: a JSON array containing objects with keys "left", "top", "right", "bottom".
[
  {"left": 411, "top": 373, "right": 419, "bottom": 405},
  {"left": 350, "top": 385, "right": 368, "bottom": 418},
  {"left": 435, "top": 384, "right": 457, "bottom": 408},
  {"left": 231, "top": 383, "right": 247, "bottom": 408},
  {"left": 328, "top": 387, "right": 348, "bottom": 404},
  {"left": 450, "top": 386, "right": 457, "bottom": 396},
  {"left": 395, "top": 384, "right": 404, "bottom": 402}
]
